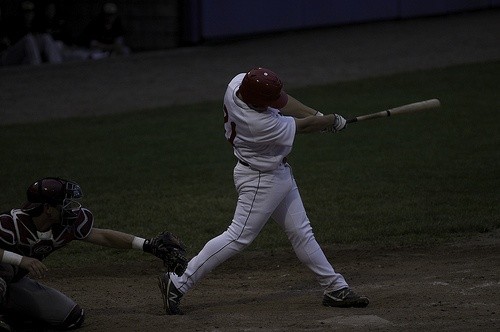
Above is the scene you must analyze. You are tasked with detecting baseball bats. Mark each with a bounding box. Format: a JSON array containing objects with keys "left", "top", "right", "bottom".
[{"left": 346, "top": 99, "right": 441, "bottom": 124}]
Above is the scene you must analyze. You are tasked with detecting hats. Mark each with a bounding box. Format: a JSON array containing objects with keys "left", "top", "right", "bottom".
[{"left": 239, "top": 67, "right": 288, "bottom": 109}]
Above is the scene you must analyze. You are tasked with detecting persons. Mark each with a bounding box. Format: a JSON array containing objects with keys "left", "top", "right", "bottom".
[
  {"left": 157, "top": 68, "right": 371, "bottom": 313},
  {"left": 0, "top": 179, "right": 187, "bottom": 332}
]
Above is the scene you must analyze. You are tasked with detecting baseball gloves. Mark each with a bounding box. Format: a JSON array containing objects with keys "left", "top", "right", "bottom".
[{"left": 149, "top": 229, "right": 189, "bottom": 277}]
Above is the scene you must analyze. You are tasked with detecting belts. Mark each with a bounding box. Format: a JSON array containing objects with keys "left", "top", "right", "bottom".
[{"left": 239, "top": 157, "right": 288, "bottom": 170}]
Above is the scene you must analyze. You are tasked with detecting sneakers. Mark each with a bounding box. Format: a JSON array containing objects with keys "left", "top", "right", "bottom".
[
  {"left": 158, "top": 272, "right": 185, "bottom": 315},
  {"left": 322, "top": 288, "right": 369, "bottom": 308}
]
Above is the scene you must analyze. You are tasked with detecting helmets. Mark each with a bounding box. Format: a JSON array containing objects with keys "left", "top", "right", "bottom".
[{"left": 27, "top": 177, "right": 80, "bottom": 207}]
